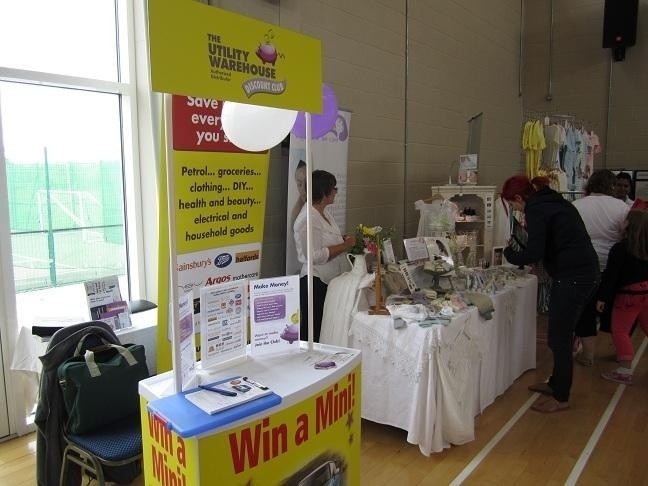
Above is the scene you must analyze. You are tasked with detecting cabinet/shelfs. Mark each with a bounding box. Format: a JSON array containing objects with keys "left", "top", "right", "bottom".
[{"left": 431, "top": 185, "right": 497, "bottom": 263}]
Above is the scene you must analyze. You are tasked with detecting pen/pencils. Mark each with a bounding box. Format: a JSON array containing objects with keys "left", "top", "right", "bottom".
[{"left": 198, "top": 382, "right": 237, "bottom": 397}]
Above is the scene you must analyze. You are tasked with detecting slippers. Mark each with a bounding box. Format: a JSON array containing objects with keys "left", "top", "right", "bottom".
[
  {"left": 526, "top": 376, "right": 558, "bottom": 393},
  {"left": 529, "top": 393, "right": 573, "bottom": 414}
]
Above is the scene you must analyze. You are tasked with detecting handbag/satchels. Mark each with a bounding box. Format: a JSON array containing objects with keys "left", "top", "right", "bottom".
[
  {"left": 56, "top": 331, "right": 151, "bottom": 438},
  {"left": 541, "top": 245, "right": 616, "bottom": 311}
]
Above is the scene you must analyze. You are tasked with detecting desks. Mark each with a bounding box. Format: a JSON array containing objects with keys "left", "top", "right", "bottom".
[
  {"left": 316, "top": 269, "right": 537, "bottom": 457},
  {"left": 137, "top": 341, "right": 361, "bottom": 486}
]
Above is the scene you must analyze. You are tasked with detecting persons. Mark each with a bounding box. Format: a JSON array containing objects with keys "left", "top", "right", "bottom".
[
  {"left": 571, "top": 169, "right": 631, "bottom": 367},
  {"left": 293, "top": 169, "right": 356, "bottom": 342},
  {"left": 291, "top": 160, "right": 306, "bottom": 232},
  {"left": 501, "top": 175, "right": 589, "bottom": 410},
  {"left": 614, "top": 172, "right": 635, "bottom": 206},
  {"left": 600, "top": 198, "right": 648, "bottom": 385}
]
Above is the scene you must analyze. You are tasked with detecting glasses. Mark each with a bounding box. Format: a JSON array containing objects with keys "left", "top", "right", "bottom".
[{"left": 331, "top": 185, "right": 339, "bottom": 191}]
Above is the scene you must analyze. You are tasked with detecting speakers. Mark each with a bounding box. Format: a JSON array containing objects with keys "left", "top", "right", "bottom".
[{"left": 601, "top": 0, "right": 639, "bottom": 48}]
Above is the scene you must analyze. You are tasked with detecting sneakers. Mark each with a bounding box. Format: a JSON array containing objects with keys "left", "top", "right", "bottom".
[
  {"left": 573, "top": 351, "right": 594, "bottom": 368},
  {"left": 597, "top": 368, "right": 633, "bottom": 387}
]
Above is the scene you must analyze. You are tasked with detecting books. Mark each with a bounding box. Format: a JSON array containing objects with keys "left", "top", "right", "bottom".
[{"left": 184, "top": 377, "right": 274, "bottom": 415}]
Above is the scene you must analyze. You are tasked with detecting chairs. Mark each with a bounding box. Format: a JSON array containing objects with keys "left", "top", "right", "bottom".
[{"left": 46, "top": 323, "right": 144, "bottom": 486}]
